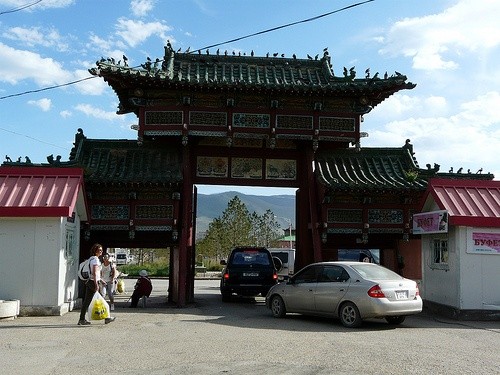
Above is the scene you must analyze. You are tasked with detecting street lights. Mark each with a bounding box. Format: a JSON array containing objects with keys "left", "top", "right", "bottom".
[{"left": 274, "top": 215, "right": 292, "bottom": 250}]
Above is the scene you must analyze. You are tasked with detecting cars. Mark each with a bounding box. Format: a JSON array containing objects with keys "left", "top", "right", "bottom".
[{"left": 264, "top": 260, "right": 423, "bottom": 329}]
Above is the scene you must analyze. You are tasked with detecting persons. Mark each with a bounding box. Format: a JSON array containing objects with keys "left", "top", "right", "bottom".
[
  {"left": 100, "top": 253, "right": 116, "bottom": 312},
  {"left": 78, "top": 243, "right": 116, "bottom": 325},
  {"left": 129, "top": 270, "right": 153, "bottom": 308}
]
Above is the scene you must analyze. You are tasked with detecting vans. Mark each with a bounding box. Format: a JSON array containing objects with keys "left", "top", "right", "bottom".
[{"left": 267, "top": 248, "right": 295, "bottom": 283}]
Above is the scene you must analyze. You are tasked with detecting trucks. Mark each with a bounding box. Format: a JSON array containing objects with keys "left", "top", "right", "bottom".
[{"left": 115, "top": 249, "right": 130, "bottom": 267}]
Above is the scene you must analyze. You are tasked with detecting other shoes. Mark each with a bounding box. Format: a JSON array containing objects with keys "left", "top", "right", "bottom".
[
  {"left": 77, "top": 319, "right": 92, "bottom": 325},
  {"left": 105, "top": 317, "right": 117, "bottom": 324},
  {"left": 111, "top": 304, "right": 115, "bottom": 310}
]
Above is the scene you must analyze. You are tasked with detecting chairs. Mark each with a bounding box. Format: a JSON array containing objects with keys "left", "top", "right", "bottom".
[{"left": 235, "top": 256, "right": 244, "bottom": 262}]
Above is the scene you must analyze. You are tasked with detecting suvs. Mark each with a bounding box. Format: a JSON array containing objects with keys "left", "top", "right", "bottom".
[{"left": 219, "top": 245, "right": 280, "bottom": 304}]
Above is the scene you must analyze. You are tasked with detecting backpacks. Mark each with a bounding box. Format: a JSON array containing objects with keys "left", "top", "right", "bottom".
[{"left": 78, "top": 256, "right": 94, "bottom": 280}]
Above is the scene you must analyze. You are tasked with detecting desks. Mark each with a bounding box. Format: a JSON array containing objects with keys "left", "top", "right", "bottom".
[{"left": 195, "top": 266, "right": 207, "bottom": 277}]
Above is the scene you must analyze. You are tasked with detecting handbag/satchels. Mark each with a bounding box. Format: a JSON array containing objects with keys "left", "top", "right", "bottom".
[{"left": 87, "top": 290, "right": 110, "bottom": 321}]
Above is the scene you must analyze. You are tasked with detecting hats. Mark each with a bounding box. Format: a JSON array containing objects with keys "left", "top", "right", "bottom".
[{"left": 139, "top": 270, "right": 148, "bottom": 276}]
